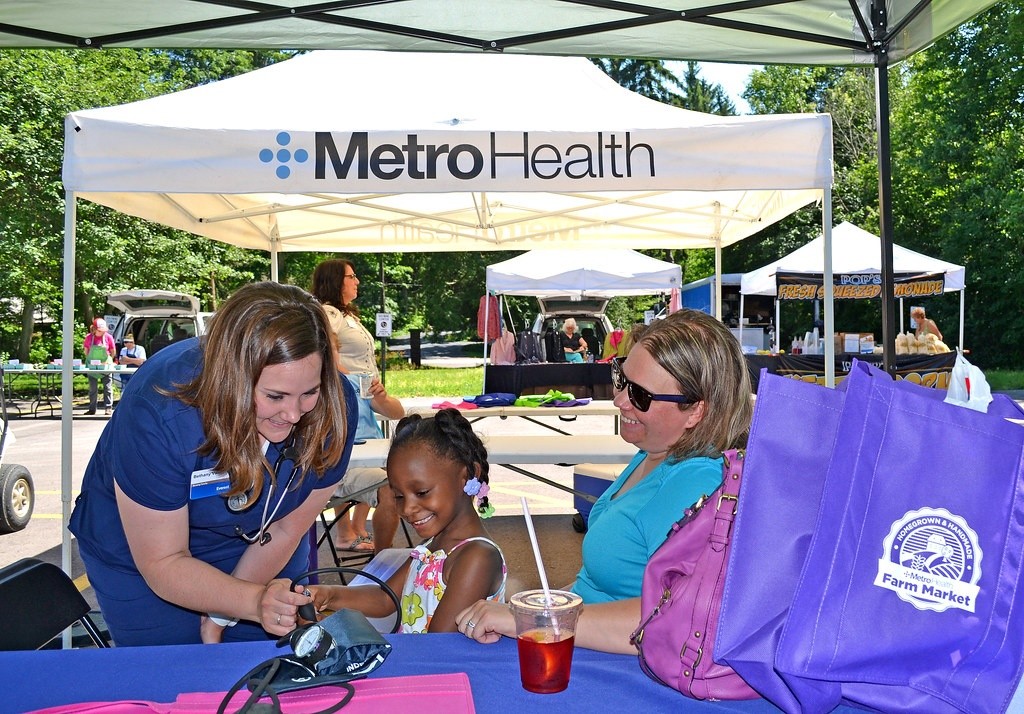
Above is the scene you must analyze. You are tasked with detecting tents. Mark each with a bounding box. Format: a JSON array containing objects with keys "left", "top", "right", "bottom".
[
  {"left": 483, "top": 248, "right": 682, "bottom": 397},
  {"left": 62, "top": 53, "right": 835, "bottom": 648},
  {"left": 738, "top": 222, "right": 965, "bottom": 356}
]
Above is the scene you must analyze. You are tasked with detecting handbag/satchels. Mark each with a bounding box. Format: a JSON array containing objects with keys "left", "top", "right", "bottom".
[
  {"left": 629, "top": 448, "right": 761, "bottom": 700},
  {"left": 712, "top": 357, "right": 1024, "bottom": 714}
]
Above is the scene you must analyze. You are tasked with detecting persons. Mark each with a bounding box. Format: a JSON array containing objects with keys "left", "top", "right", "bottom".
[
  {"left": 559, "top": 318, "right": 586, "bottom": 362},
  {"left": 455, "top": 309, "right": 751, "bottom": 655},
  {"left": 83, "top": 318, "right": 116, "bottom": 415},
  {"left": 313, "top": 257, "right": 381, "bottom": 552},
  {"left": 912, "top": 308, "right": 942, "bottom": 341},
  {"left": 68, "top": 280, "right": 358, "bottom": 648},
  {"left": 302, "top": 409, "right": 506, "bottom": 633},
  {"left": 119, "top": 334, "right": 147, "bottom": 391},
  {"left": 333, "top": 347, "right": 404, "bottom": 559},
  {"left": 757, "top": 301, "right": 772, "bottom": 330}
]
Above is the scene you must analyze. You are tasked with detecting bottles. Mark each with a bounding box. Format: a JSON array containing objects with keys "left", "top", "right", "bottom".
[{"left": 791, "top": 336, "right": 803, "bottom": 355}]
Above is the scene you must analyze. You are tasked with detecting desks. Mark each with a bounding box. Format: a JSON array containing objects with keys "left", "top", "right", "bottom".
[
  {"left": 1, "top": 368, "right": 138, "bottom": 418},
  {"left": 483, "top": 363, "right": 614, "bottom": 399},
  {"left": 348, "top": 434, "right": 641, "bottom": 469},
  {"left": 374, "top": 398, "right": 625, "bottom": 439},
  {"left": 0, "top": 629, "right": 789, "bottom": 714},
  {"left": 742, "top": 351, "right": 957, "bottom": 395}
]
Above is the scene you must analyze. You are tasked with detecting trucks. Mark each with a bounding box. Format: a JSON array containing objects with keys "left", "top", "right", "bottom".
[{"left": 654, "top": 273, "right": 775, "bottom": 351}]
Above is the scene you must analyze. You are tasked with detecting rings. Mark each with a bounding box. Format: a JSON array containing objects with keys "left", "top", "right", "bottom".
[
  {"left": 278, "top": 614, "right": 282, "bottom": 624},
  {"left": 468, "top": 621, "right": 475, "bottom": 628}
]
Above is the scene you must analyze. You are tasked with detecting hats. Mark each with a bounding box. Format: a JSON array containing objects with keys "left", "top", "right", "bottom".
[
  {"left": 93, "top": 318, "right": 109, "bottom": 332},
  {"left": 123, "top": 334, "right": 134, "bottom": 343}
]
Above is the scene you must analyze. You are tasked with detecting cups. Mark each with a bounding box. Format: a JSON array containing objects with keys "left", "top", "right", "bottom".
[
  {"left": 359, "top": 373, "right": 375, "bottom": 400},
  {"left": 508, "top": 589, "right": 583, "bottom": 694}
]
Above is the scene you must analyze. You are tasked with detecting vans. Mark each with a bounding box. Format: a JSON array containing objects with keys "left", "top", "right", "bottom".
[
  {"left": 106, "top": 290, "right": 214, "bottom": 362},
  {"left": 534, "top": 295, "right": 614, "bottom": 363}
]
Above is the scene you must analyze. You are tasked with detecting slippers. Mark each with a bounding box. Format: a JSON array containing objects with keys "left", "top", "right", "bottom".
[
  {"left": 358, "top": 531, "right": 374, "bottom": 541},
  {"left": 335, "top": 536, "right": 373, "bottom": 552}
]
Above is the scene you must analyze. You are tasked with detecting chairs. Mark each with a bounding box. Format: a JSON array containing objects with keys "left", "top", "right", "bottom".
[
  {"left": 173, "top": 329, "right": 188, "bottom": 344},
  {"left": 544, "top": 328, "right": 559, "bottom": 355},
  {"left": 150, "top": 336, "right": 168, "bottom": 355},
  {"left": 0, "top": 558, "right": 112, "bottom": 651},
  {"left": 581, "top": 328, "right": 599, "bottom": 356}
]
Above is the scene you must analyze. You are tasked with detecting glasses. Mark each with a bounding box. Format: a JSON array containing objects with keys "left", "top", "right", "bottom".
[
  {"left": 611, "top": 356, "right": 701, "bottom": 412},
  {"left": 343, "top": 274, "right": 356, "bottom": 280},
  {"left": 124, "top": 341, "right": 132, "bottom": 343}
]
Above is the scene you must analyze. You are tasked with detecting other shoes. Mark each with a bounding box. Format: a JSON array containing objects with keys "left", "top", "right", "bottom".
[
  {"left": 105, "top": 408, "right": 112, "bottom": 415},
  {"left": 84, "top": 410, "right": 95, "bottom": 415}
]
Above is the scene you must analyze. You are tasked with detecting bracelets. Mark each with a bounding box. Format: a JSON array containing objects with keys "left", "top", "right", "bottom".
[{"left": 209, "top": 613, "right": 239, "bottom": 626}]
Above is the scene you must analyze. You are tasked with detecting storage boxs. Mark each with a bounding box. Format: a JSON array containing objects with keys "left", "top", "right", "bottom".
[
  {"left": 4, "top": 364, "right": 15, "bottom": 369},
  {"left": 47, "top": 364, "right": 55, "bottom": 369},
  {"left": 572, "top": 464, "right": 630, "bottom": 528},
  {"left": 97, "top": 365, "right": 108, "bottom": 370},
  {"left": 55, "top": 366, "right": 62, "bottom": 370},
  {"left": 74, "top": 365, "right": 85, "bottom": 370},
  {"left": 114, "top": 365, "right": 127, "bottom": 370},
  {"left": 834, "top": 333, "right": 874, "bottom": 355},
  {"left": 88, "top": 364, "right": 97, "bottom": 369},
  {"left": 16, "top": 364, "right": 33, "bottom": 369}
]
